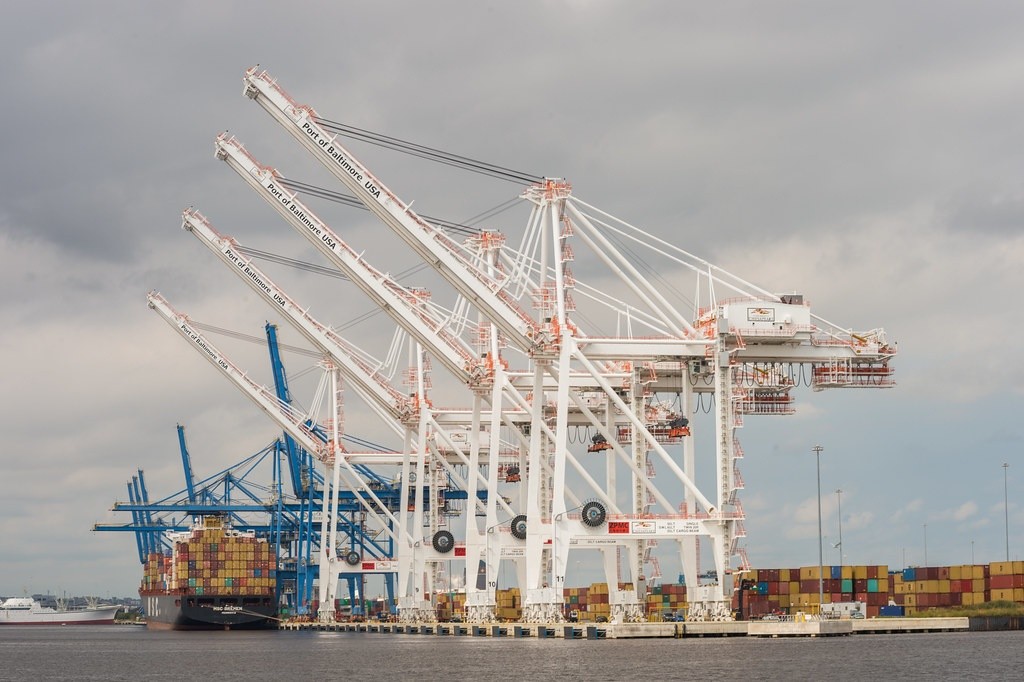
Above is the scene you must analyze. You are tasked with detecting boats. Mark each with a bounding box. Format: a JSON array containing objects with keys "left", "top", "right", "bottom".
[
  {"left": 136, "top": 513, "right": 278, "bottom": 631},
  {"left": 0, "top": 588, "right": 122, "bottom": 625}
]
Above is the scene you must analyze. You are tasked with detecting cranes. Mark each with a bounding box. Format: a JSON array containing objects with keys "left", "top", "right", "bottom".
[
  {"left": 89, "top": 127, "right": 799, "bottom": 620},
  {"left": 241, "top": 62, "right": 902, "bottom": 623}
]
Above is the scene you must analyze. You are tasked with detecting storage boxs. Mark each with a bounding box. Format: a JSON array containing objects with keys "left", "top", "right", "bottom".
[{"left": 141, "top": 529, "right": 1024, "bottom": 621}]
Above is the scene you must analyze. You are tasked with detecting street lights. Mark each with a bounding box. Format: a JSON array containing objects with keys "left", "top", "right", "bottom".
[
  {"left": 834, "top": 489, "right": 845, "bottom": 565},
  {"left": 971, "top": 540, "right": 975, "bottom": 565},
  {"left": 813, "top": 444, "right": 826, "bottom": 613},
  {"left": 1001, "top": 462, "right": 1011, "bottom": 560},
  {"left": 921, "top": 523, "right": 928, "bottom": 566}
]
